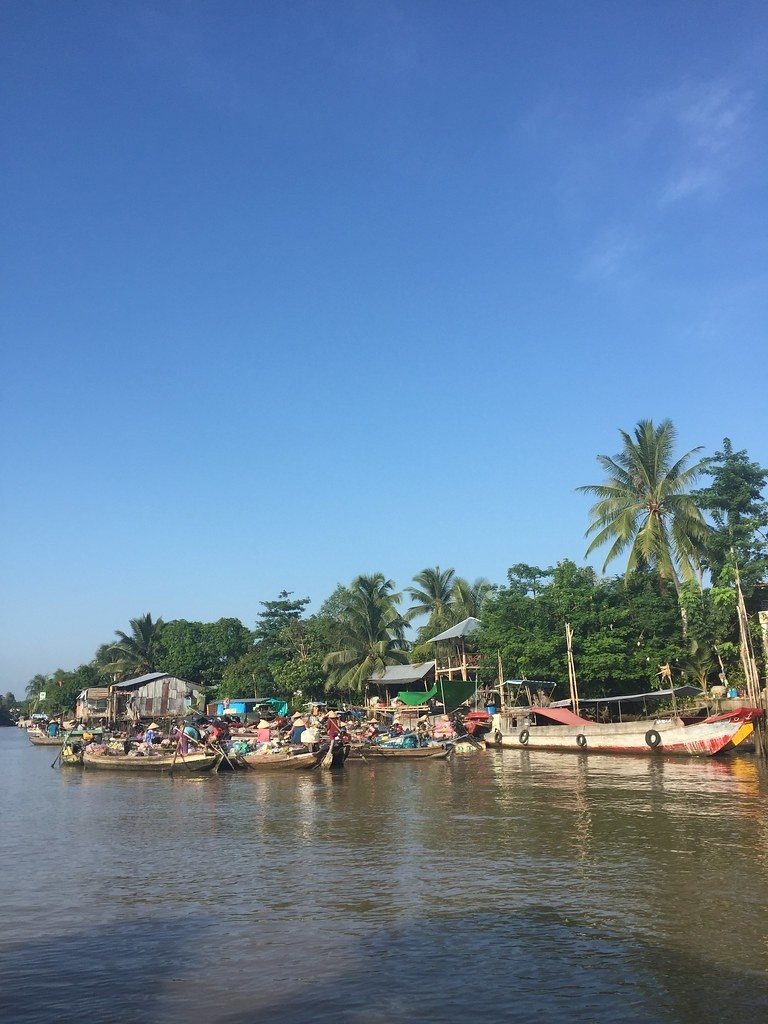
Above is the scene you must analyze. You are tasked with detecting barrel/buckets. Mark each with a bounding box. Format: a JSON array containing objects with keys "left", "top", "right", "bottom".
[
  {"left": 485, "top": 706, "right": 495, "bottom": 715},
  {"left": 727, "top": 690, "right": 737, "bottom": 698}
]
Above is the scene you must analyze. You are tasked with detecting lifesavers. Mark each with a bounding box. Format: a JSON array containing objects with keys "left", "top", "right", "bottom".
[
  {"left": 519, "top": 730, "right": 529, "bottom": 743},
  {"left": 576, "top": 734, "right": 586, "bottom": 746},
  {"left": 495, "top": 732, "right": 502, "bottom": 743},
  {"left": 645, "top": 729, "right": 661, "bottom": 747}
]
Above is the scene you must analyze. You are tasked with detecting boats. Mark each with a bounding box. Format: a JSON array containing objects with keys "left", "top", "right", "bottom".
[
  {"left": 344, "top": 740, "right": 456, "bottom": 758},
  {"left": 61, "top": 741, "right": 84, "bottom": 766},
  {"left": 484, "top": 678, "right": 766, "bottom": 756},
  {"left": 83, "top": 741, "right": 218, "bottom": 770},
  {"left": 29, "top": 735, "right": 93, "bottom": 746},
  {"left": 223, "top": 742, "right": 328, "bottom": 768}
]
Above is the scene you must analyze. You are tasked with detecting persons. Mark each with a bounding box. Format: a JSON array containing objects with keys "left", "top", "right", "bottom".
[
  {"left": 322, "top": 710, "right": 338, "bottom": 738},
  {"left": 367, "top": 717, "right": 380, "bottom": 735},
  {"left": 416, "top": 711, "right": 436, "bottom": 730},
  {"left": 288, "top": 711, "right": 307, "bottom": 746},
  {"left": 48, "top": 719, "right": 58, "bottom": 738},
  {"left": 146, "top": 722, "right": 159, "bottom": 743},
  {"left": 204, "top": 717, "right": 229, "bottom": 750},
  {"left": 176, "top": 720, "right": 201, "bottom": 754},
  {"left": 258, "top": 719, "right": 272, "bottom": 742}
]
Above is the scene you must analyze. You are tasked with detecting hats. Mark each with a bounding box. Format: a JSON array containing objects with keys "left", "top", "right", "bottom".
[
  {"left": 292, "top": 718, "right": 305, "bottom": 726},
  {"left": 292, "top": 712, "right": 302, "bottom": 717},
  {"left": 395, "top": 714, "right": 401, "bottom": 719},
  {"left": 348, "top": 720, "right": 353, "bottom": 724},
  {"left": 49, "top": 720, "right": 56, "bottom": 723},
  {"left": 147, "top": 723, "right": 159, "bottom": 729},
  {"left": 341, "top": 722, "right": 347, "bottom": 726},
  {"left": 257, "top": 720, "right": 269, "bottom": 728},
  {"left": 326, "top": 711, "right": 336, "bottom": 717},
  {"left": 367, "top": 718, "right": 379, "bottom": 722}
]
